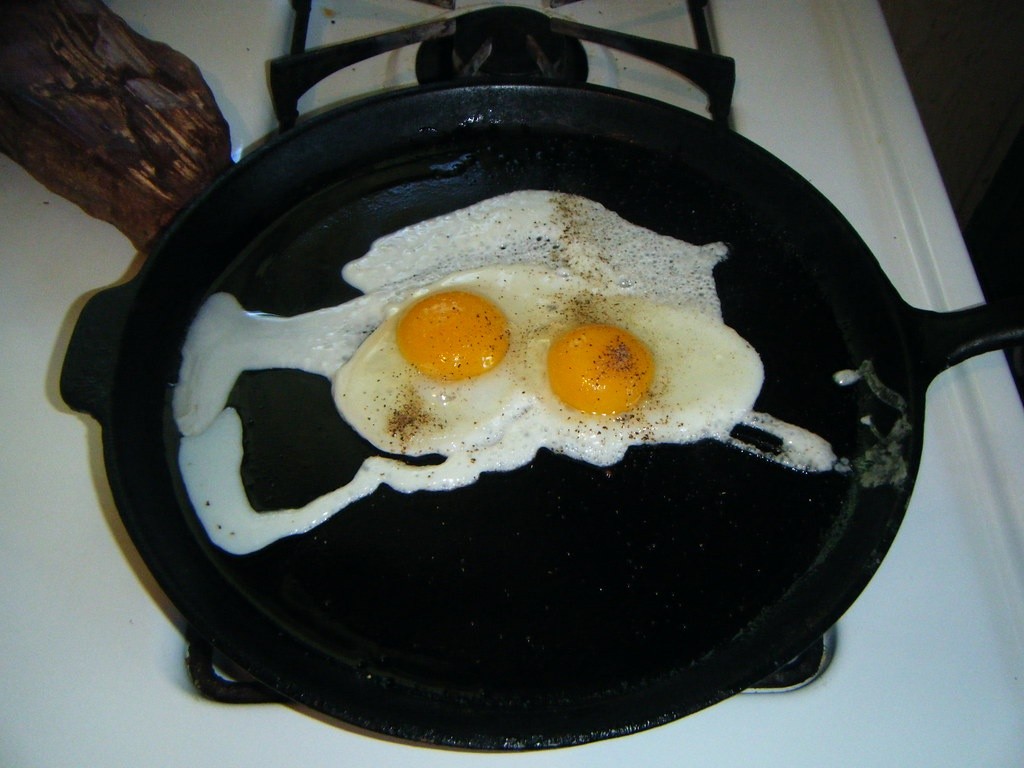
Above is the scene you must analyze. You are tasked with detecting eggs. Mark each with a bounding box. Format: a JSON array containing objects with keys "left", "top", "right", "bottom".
[
  {"left": 170, "top": 189, "right": 541, "bottom": 555},
  {"left": 530, "top": 192, "right": 839, "bottom": 472}
]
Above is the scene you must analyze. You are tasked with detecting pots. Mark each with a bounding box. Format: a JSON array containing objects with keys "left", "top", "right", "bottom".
[{"left": 59, "top": 79, "right": 1024, "bottom": 752}]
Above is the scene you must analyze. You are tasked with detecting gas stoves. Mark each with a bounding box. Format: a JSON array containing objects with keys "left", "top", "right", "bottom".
[{"left": 0, "top": 0, "right": 1024, "bottom": 767}]
